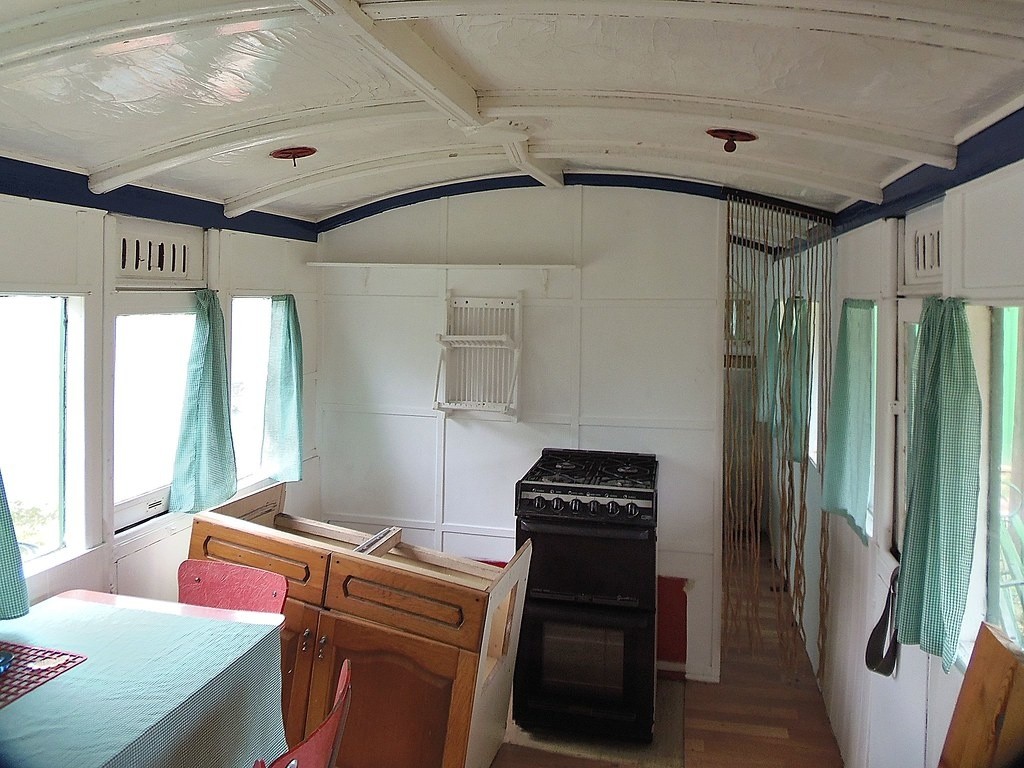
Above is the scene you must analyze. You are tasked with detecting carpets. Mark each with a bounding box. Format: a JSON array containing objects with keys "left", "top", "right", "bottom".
[{"left": 491, "top": 677, "right": 689, "bottom": 768}]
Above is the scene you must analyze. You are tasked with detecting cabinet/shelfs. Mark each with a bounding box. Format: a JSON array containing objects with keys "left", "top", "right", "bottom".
[
  {"left": 431, "top": 289, "right": 524, "bottom": 423},
  {"left": 188, "top": 481, "right": 533, "bottom": 768}
]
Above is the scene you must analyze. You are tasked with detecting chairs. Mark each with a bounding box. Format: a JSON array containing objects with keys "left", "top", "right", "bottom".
[
  {"left": 259, "top": 659, "right": 354, "bottom": 768},
  {"left": 177, "top": 559, "right": 289, "bottom": 615}
]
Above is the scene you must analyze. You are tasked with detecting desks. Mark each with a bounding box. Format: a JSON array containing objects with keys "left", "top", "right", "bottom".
[{"left": 0, "top": 589, "right": 290, "bottom": 768}]
